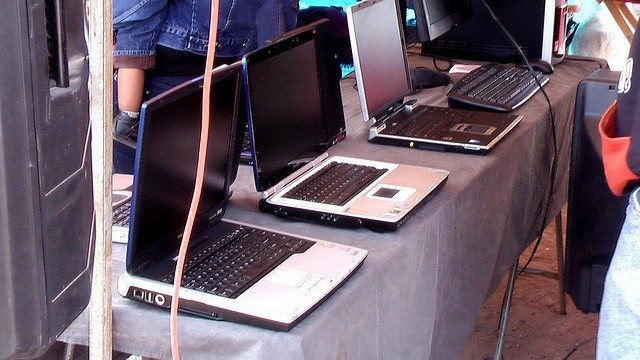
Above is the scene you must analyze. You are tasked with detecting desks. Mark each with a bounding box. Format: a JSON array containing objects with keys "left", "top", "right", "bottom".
[{"left": 55, "top": 43, "right": 610, "bottom": 359}]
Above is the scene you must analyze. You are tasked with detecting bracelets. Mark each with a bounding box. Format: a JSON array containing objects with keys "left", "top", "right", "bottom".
[{"left": 120, "top": 111, "right": 139, "bottom": 119}]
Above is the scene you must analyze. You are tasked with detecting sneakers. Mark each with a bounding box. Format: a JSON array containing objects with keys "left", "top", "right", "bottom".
[{"left": 113, "top": 110, "right": 139, "bottom": 151}]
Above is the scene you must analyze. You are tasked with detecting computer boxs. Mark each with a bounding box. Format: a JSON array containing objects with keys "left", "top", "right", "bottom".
[{"left": 417, "top": 0, "right": 567, "bottom": 65}]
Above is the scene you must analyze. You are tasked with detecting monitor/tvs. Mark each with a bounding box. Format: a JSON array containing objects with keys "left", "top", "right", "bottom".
[{"left": 395, "top": 0, "right": 461, "bottom": 91}]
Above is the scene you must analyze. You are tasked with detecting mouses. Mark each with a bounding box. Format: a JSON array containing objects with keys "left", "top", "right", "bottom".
[{"left": 515, "top": 60, "right": 555, "bottom": 75}]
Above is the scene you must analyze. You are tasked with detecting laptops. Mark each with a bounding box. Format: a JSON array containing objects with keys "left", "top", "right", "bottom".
[
  {"left": 112, "top": 60, "right": 246, "bottom": 243},
  {"left": 243, "top": 17, "right": 450, "bottom": 234},
  {"left": 346, "top": 1, "right": 523, "bottom": 155},
  {"left": 117, "top": 62, "right": 369, "bottom": 333},
  {"left": 239, "top": 18, "right": 346, "bottom": 166}
]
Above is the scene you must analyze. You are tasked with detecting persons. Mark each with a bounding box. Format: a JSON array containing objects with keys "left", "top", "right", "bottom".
[
  {"left": 82, "top": 0, "right": 300, "bottom": 176},
  {"left": 596, "top": 8, "right": 640, "bottom": 360}
]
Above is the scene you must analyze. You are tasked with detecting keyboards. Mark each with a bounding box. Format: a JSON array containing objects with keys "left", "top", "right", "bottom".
[{"left": 446, "top": 62, "right": 550, "bottom": 114}]
